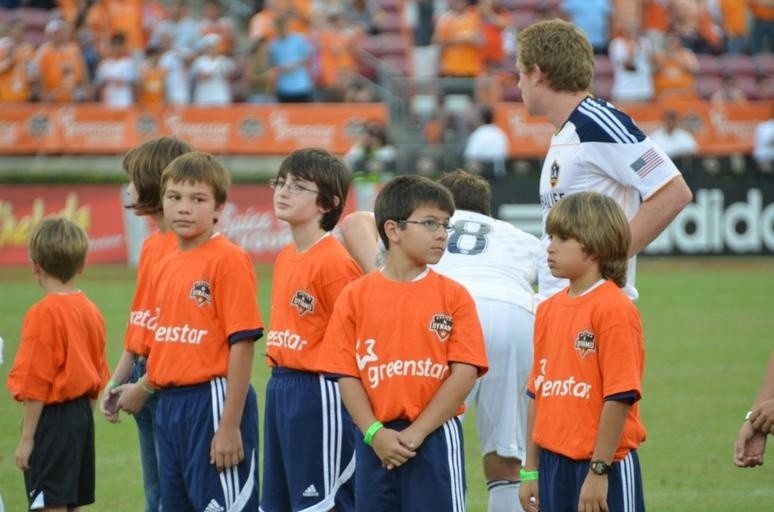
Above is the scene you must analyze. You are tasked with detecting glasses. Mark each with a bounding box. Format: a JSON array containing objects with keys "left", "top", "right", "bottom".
[
  {"left": 268, "top": 177, "right": 318, "bottom": 197},
  {"left": 399, "top": 217, "right": 455, "bottom": 234}
]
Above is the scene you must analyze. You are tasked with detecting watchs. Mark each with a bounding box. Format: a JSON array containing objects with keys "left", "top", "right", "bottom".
[{"left": 588, "top": 459, "right": 611, "bottom": 476}]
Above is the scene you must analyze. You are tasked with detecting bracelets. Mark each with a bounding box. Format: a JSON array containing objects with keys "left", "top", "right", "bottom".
[
  {"left": 518, "top": 468, "right": 539, "bottom": 481},
  {"left": 137, "top": 376, "right": 156, "bottom": 395},
  {"left": 745, "top": 410, "right": 753, "bottom": 420},
  {"left": 106, "top": 380, "right": 120, "bottom": 389},
  {"left": 363, "top": 421, "right": 384, "bottom": 446}
]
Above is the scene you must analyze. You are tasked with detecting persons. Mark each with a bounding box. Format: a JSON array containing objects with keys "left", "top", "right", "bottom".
[
  {"left": 145, "top": 154, "right": 265, "bottom": 512},
  {"left": 8, "top": 215, "right": 111, "bottom": 512},
  {"left": 732, "top": 355, "right": 774, "bottom": 468},
  {"left": 96, "top": 135, "right": 188, "bottom": 512},
  {"left": 516, "top": 19, "right": 693, "bottom": 303},
  {"left": 517, "top": 190, "right": 648, "bottom": 512},
  {"left": 341, "top": 167, "right": 542, "bottom": 512},
  {"left": 317, "top": 172, "right": 488, "bottom": 512},
  {"left": 258, "top": 148, "right": 366, "bottom": 512}
]
